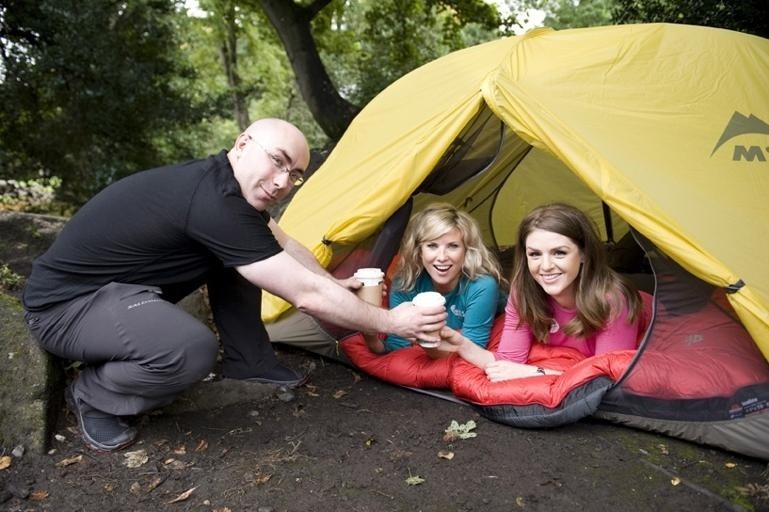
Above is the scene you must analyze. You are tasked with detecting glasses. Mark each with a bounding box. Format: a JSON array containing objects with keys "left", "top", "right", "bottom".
[{"left": 248, "top": 135, "right": 305, "bottom": 186}]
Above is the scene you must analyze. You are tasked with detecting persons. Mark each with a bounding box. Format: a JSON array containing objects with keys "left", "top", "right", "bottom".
[{"left": 25, "top": 118, "right": 448, "bottom": 451}]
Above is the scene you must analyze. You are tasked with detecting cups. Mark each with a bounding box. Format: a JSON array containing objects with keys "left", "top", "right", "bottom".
[
  {"left": 413, "top": 290, "right": 447, "bottom": 349},
  {"left": 354, "top": 267, "right": 385, "bottom": 308}
]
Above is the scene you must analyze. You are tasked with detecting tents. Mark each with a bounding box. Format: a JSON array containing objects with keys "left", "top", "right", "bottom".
[{"left": 259, "top": 23, "right": 769, "bottom": 458}]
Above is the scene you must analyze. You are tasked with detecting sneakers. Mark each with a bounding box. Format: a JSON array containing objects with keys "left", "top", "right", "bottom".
[
  {"left": 222, "top": 359, "right": 305, "bottom": 384},
  {"left": 69, "top": 378, "right": 137, "bottom": 453}
]
